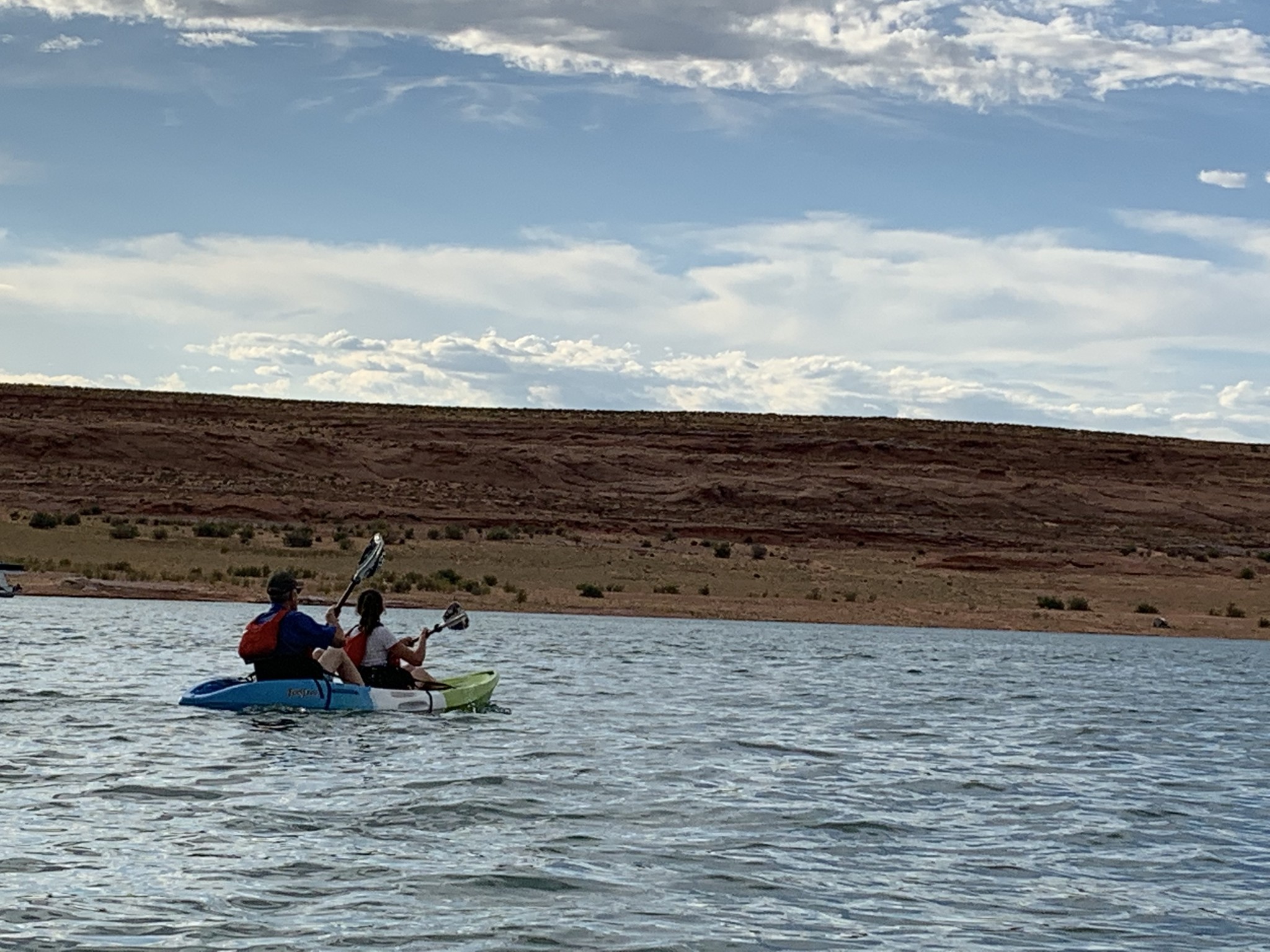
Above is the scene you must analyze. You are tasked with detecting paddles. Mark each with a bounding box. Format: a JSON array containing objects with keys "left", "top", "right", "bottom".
[
  {"left": 409, "top": 601, "right": 470, "bottom": 643},
  {"left": 325, "top": 532, "right": 386, "bottom": 628}
]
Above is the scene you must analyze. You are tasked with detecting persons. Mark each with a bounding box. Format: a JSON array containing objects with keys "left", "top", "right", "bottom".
[
  {"left": 344, "top": 589, "right": 436, "bottom": 689},
  {"left": 253, "top": 571, "right": 366, "bottom": 686}
]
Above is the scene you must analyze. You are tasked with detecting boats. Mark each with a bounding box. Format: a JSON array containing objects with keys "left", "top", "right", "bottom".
[{"left": 179, "top": 668, "right": 499, "bottom": 714}]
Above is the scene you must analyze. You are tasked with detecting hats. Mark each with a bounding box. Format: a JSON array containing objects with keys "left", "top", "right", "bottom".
[{"left": 266, "top": 571, "right": 304, "bottom": 595}]
